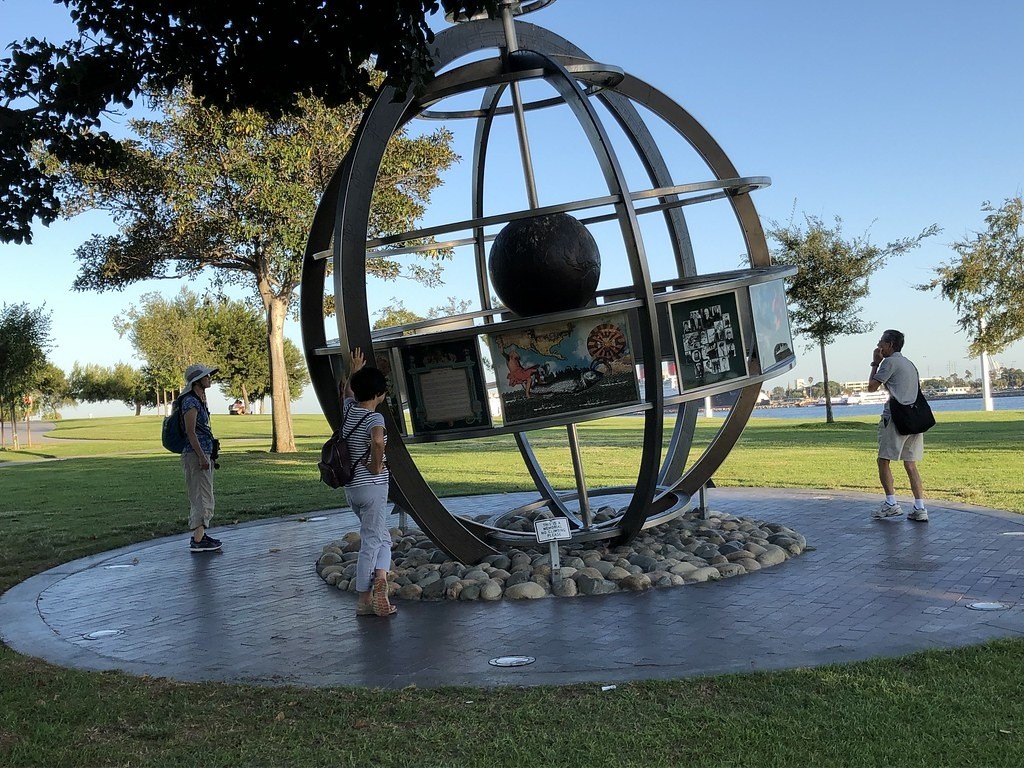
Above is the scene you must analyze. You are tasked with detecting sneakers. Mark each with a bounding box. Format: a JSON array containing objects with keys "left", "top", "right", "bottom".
[
  {"left": 189, "top": 533, "right": 223, "bottom": 551},
  {"left": 870, "top": 501, "right": 904, "bottom": 520},
  {"left": 907, "top": 506, "right": 929, "bottom": 520}
]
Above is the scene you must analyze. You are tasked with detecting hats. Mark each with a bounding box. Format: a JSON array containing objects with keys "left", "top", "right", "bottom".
[{"left": 176, "top": 363, "right": 220, "bottom": 400}]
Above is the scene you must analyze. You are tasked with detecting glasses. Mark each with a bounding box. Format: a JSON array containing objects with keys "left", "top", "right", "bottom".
[{"left": 204, "top": 373, "right": 211, "bottom": 378}]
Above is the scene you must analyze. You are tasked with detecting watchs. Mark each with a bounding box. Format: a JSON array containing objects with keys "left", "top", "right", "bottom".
[{"left": 870, "top": 362, "right": 879, "bottom": 367}]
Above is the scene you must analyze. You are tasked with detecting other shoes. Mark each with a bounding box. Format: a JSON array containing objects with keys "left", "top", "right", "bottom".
[
  {"left": 371, "top": 575, "right": 390, "bottom": 617},
  {"left": 355, "top": 600, "right": 398, "bottom": 615}
]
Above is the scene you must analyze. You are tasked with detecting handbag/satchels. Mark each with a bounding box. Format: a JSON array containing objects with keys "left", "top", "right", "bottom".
[{"left": 889, "top": 389, "right": 937, "bottom": 436}]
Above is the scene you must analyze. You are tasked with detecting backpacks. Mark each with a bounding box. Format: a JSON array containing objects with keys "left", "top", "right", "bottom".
[
  {"left": 318, "top": 405, "right": 385, "bottom": 489},
  {"left": 161, "top": 391, "right": 202, "bottom": 453}
]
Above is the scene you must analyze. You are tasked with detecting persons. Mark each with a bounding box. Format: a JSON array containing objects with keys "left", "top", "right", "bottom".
[
  {"left": 683, "top": 305, "right": 736, "bottom": 378},
  {"left": 230, "top": 400, "right": 253, "bottom": 415},
  {"left": 868, "top": 329, "right": 929, "bottom": 522},
  {"left": 177, "top": 364, "right": 224, "bottom": 551},
  {"left": 342, "top": 347, "right": 398, "bottom": 616}
]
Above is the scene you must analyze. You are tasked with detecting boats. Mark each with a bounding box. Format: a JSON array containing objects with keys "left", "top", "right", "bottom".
[
  {"left": 815, "top": 397, "right": 848, "bottom": 406},
  {"left": 847, "top": 390, "right": 891, "bottom": 405}
]
